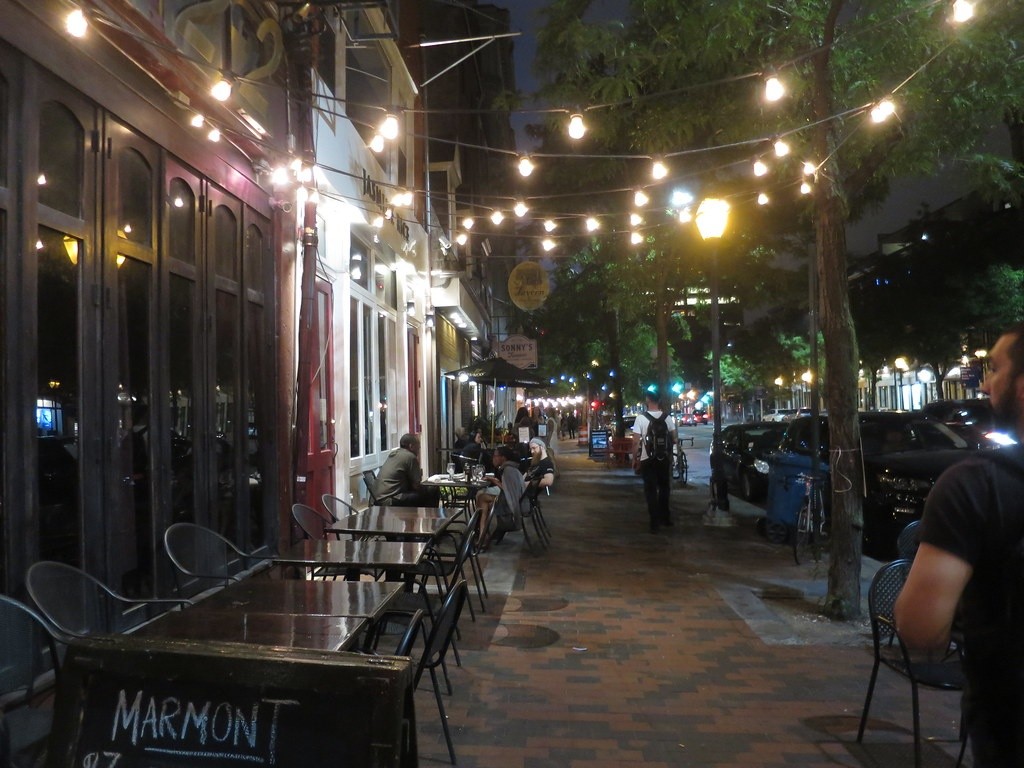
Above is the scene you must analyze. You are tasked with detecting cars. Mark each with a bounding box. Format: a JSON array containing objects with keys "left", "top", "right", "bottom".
[
  {"left": 710, "top": 400, "right": 1020, "bottom": 562},
  {"left": 671, "top": 408, "right": 708, "bottom": 427}
]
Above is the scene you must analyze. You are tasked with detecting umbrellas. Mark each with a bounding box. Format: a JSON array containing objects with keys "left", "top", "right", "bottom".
[{"left": 444, "top": 356, "right": 551, "bottom": 445}]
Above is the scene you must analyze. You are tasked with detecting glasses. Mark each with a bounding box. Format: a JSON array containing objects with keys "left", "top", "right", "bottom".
[{"left": 491, "top": 453, "right": 503, "bottom": 458}]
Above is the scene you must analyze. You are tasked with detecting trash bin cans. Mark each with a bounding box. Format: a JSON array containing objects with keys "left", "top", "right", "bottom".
[
  {"left": 755, "top": 454, "right": 829, "bottom": 544},
  {"left": 578, "top": 426, "right": 588, "bottom": 445}
]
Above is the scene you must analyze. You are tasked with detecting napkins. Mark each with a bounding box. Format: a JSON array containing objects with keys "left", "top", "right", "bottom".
[{"left": 428, "top": 477, "right": 441, "bottom": 482}]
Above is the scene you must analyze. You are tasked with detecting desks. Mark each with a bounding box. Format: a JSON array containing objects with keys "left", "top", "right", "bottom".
[
  {"left": 188, "top": 578, "right": 406, "bottom": 651},
  {"left": 272, "top": 538, "right": 429, "bottom": 651},
  {"left": 421, "top": 473, "right": 491, "bottom": 525},
  {"left": 323, "top": 506, "right": 464, "bottom": 632},
  {"left": 130, "top": 610, "right": 366, "bottom": 652}
]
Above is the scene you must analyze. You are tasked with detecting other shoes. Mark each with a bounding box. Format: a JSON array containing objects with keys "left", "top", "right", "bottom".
[
  {"left": 649, "top": 525, "right": 660, "bottom": 534},
  {"left": 473, "top": 542, "right": 489, "bottom": 552},
  {"left": 658, "top": 516, "right": 674, "bottom": 527}
]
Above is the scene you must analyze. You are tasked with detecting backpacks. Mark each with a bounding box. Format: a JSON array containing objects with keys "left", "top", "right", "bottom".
[
  {"left": 642, "top": 411, "right": 673, "bottom": 465},
  {"left": 971, "top": 449, "right": 1024, "bottom": 680}
]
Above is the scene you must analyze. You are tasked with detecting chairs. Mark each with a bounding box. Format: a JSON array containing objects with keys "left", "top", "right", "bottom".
[
  {"left": 882, "top": 431, "right": 907, "bottom": 449},
  {"left": 0, "top": 494, "right": 489, "bottom": 768},
  {"left": 362, "top": 470, "right": 381, "bottom": 507},
  {"left": 452, "top": 454, "right": 478, "bottom": 475},
  {"left": 475, "top": 476, "right": 551, "bottom": 554},
  {"left": 856, "top": 521, "right": 971, "bottom": 768}
]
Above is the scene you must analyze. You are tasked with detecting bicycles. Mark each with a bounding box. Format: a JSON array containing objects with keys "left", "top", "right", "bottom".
[
  {"left": 672, "top": 437, "right": 694, "bottom": 484},
  {"left": 793, "top": 474, "right": 828, "bottom": 565}
]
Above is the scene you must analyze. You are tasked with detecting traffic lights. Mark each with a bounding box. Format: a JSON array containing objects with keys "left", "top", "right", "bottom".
[
  {"left": 590, "top": 400, "right": 606, "bottom": 412},
  {"left": 706, "top": 395, "right": 713, "bottom": 404},
  {"left": 671, "top": 378, "right": 683, "bottom": 397},
  {"left": 646, "top": 380, "right": 660, "bottom": 400}
]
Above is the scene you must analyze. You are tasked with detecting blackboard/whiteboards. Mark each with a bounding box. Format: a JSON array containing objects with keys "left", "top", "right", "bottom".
[{"left": 44, "top": 637, "right": 420, "bottom": 768}]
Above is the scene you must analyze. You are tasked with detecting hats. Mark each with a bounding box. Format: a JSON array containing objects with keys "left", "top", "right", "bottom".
[{"left": 530, "top": 436, "right": 547, "bottom": 452}]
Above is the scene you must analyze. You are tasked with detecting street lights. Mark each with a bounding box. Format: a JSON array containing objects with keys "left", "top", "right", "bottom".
[
  {"left": 918, "top": 369, "right": 931, "bottom": 405},
  {"left": 895, "top": 358, "right": 906, "bottom": 411},
  {"left": 976, "top": 350, "right": 987, "bottom": 384},
  {"left": 696, "top": 199, "right": 738, "bottom": 528}
]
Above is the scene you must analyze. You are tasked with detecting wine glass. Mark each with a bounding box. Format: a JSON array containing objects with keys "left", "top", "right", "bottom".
[
  {"left": 447, "top": 463, "right": 455, "bottom": 480},
  {"left": 472, "top": 464, "right": 485, "bottom": 485}
]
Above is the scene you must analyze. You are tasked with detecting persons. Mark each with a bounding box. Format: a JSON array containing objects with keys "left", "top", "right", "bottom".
[
  {"left": 555, "top": 411, "right": 577, "bottom": 439},
  {"left": 631, "top": 390, "right": 677, "bottom": 536},
  {"left": 451, "top": 426, "right": 491, "bottom": 474},
  {"left": 475, "top": 436, "right": 554, "bottom": 547},
  {"left": 374, "top": 433, "right": 439, "bottom": 508},
  {"left": 130, "top": 403, "right": 150, "bottom": 456},
  {"left": 895, "top": 322, "right": 1024, "bottom": 768},
  {"left": 513, "top": 406, "right": 560, "bottom": 469}
]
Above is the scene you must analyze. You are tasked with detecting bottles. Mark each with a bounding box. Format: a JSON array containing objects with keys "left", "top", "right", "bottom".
[{"left": 464, "top": 463, "right": 472, "bottom": 483}]
